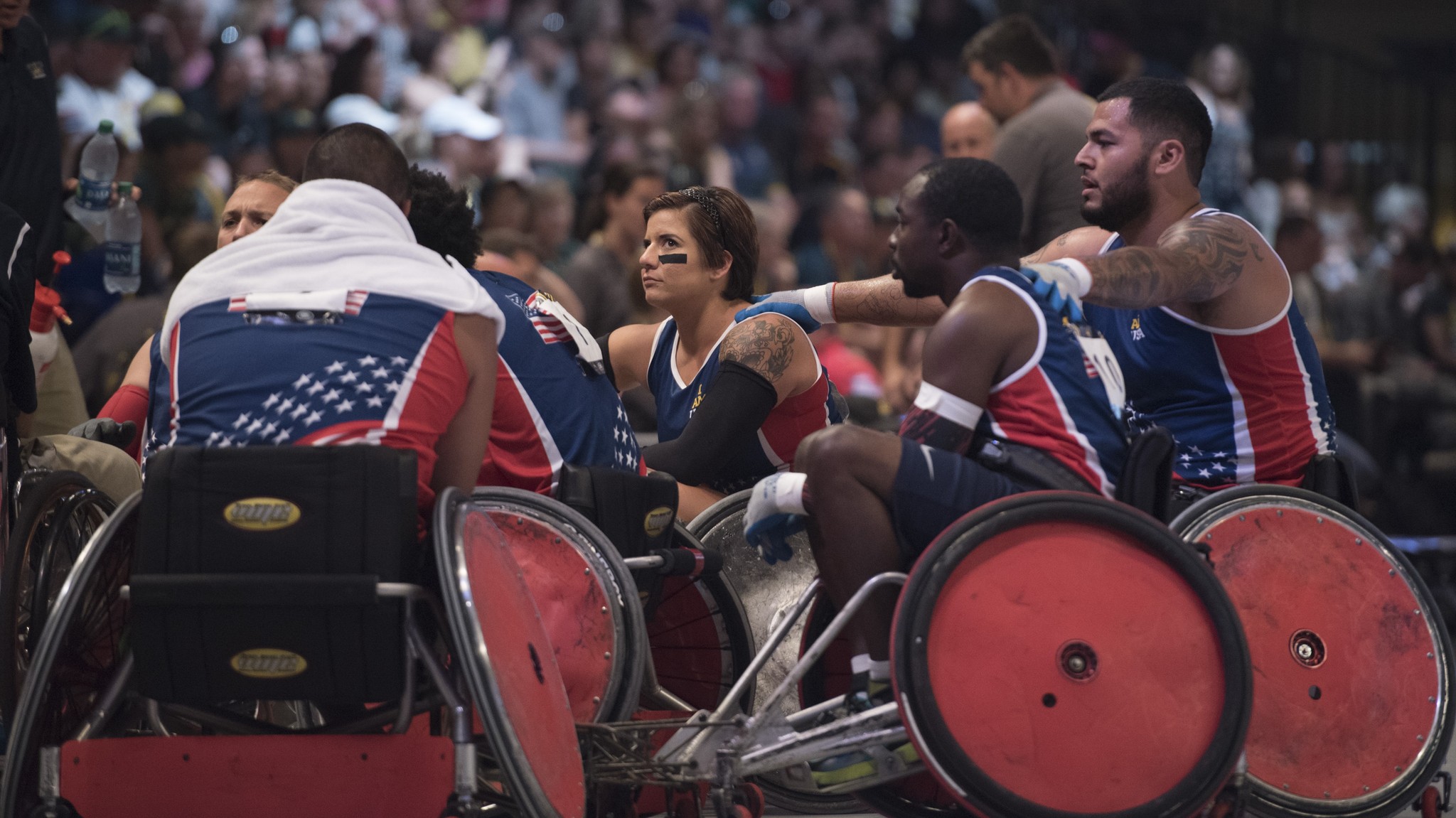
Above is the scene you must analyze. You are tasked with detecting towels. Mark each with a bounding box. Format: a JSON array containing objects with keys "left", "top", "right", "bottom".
[{"left": 158, "top": 176, "right": 508, "bottom": 375}]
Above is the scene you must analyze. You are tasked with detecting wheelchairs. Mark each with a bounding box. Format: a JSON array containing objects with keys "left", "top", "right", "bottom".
[{"left": 1, "top": 407, "right": 1456, "bottom": 818}]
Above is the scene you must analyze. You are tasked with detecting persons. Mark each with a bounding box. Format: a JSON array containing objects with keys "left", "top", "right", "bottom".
[
  {"left": 0, "top": 0, "right": 1456, "bottom": 818},
  {"left": 731, "top": 157, "right": 1124, "bottom": 791}
]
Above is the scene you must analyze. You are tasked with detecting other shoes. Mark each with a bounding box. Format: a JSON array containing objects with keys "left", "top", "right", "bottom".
[{"left": 781, "top": 686, "right": 923, "bottom": 785}]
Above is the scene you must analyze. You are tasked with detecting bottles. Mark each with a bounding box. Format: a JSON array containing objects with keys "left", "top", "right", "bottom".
[
  {"left": 73, "top": 121, "right": 118, "bottom": 226},
  {"left": 104, "top": 181, "right": 142, "bottom": 294}
]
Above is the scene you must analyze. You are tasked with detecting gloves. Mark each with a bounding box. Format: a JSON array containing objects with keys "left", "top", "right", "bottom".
[
  {"left": 742, "top": 472, "right": 812, "bottom": 549},
  {"left": 733, "top": 281, "right": 839, "bottom": 333},
  {"left": 67, "top": 417, "right": 138, "bottom": 450},
  {"left": 1020, "top": 258, "right": 1092, "bottom": 322},
  {"left": 758, "top": 515, "right": 808, "bottom": 566}
]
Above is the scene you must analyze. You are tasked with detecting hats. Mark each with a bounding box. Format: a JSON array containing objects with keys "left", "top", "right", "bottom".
[
  {"left": 421, "top": 95, "right": 506, "bottom": 142},
  {"left": 137, "top": 86, "right": 224, "bottom": 151},
  {"left": 323, "top": 93, "right": 404, "bottom": 135},
  {"left": 81, "top": 6, "right": 145, "bottom": 45}
]
